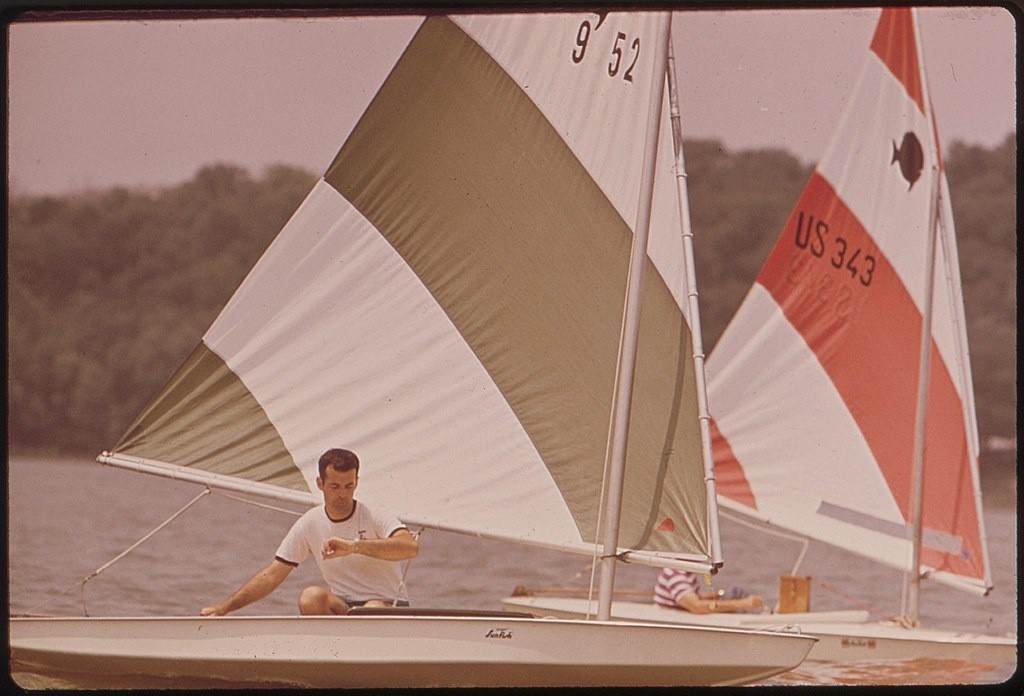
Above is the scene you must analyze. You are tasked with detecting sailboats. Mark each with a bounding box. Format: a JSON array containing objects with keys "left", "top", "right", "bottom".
[{"left": 3, "top": 10, "right": 1019, "bottom": 691}]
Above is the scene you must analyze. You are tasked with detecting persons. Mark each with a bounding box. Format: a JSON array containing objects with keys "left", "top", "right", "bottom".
[
  {"left": 200, "top": 448, "right": 420, "bottom": 617},
  {"left": 652, "top": 565, "right": 778, "bottom": 615}
]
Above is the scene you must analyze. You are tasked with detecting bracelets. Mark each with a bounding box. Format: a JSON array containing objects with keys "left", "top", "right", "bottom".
[
  {"left": 709, "top": 600, "right": 717, "bottom": 613},
  {"left": 353, "top": 538, "right": 360, "bottom": 555}
]
